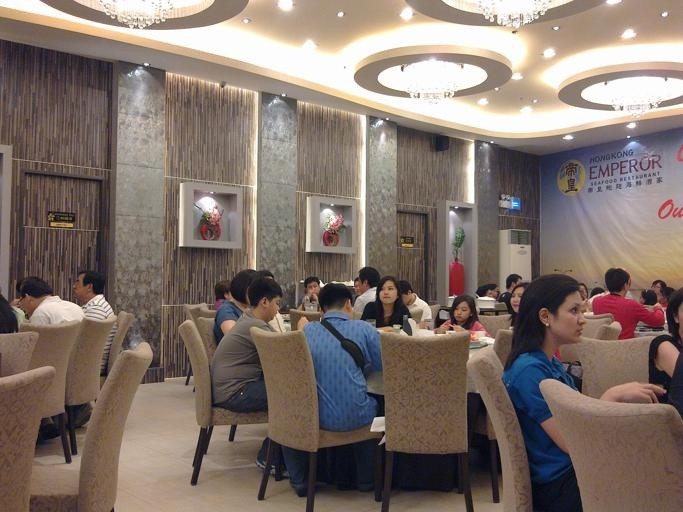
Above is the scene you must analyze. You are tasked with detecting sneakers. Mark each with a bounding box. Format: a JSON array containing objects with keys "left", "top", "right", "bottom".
[
  {"left": 71, "top": 402, "right": 93, "bottom": 427},
  {"left": 255, "top": 459, "right": 290, "bottom": 478}
]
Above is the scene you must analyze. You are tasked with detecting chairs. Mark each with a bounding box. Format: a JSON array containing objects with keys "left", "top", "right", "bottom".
[
  {"left": 177, "top": 289, "right": 683, "bottom": 512},
  {"left": 0, "top": 311, "right": 154, "bottom": 511}
]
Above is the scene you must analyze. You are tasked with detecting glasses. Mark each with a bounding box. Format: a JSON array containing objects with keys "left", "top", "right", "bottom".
[{"left": 16, "top": 295, "right": 26, "bottom": 308}]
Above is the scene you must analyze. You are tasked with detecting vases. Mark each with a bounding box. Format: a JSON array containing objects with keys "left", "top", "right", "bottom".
[
  {"left": 200, "top": 224, "right": 222, "bottom": 240},
  {"left": 323, "top": 230, "right": 339, "bottom": 247}
]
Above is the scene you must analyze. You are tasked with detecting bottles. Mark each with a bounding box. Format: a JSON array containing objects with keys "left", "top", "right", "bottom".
[{"left": 393, "top": 324, "right": 401, "bottom": 335}]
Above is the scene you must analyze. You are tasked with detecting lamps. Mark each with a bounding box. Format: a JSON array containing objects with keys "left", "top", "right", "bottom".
[
  {"left": 472, "top": 0, "right": 554, "bottom": 31},
  {"left": 604, "top": 79, "right": 667, "bottom": 121},
  {"left": 498, "top": 193, "right": 512, "bottom": 209},
  {"left": 400, "top": 60, "right": 462, "bottom": 106},
  {"left": 98, "top": 0, "right": 174, "bottom": 31}
]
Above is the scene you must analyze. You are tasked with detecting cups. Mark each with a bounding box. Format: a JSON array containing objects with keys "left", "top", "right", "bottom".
[
  {"left": 366, "top": 319, "right": 376, "bottom": 328},
  {"left": 305, "top": 303, "right": 318, "bottom": 312},
  {"left": 446, "top": 330, "right": 456, "bottom": 335}
]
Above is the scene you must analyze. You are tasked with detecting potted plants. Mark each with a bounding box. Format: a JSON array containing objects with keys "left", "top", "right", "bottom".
[{"left": 449, "top": 224, "right": 465, "bottom": 296}]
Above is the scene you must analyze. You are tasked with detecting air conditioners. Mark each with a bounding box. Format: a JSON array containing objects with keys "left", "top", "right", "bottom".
[{"left": 498, "top": 229, "right": 533, "bottom": 294}]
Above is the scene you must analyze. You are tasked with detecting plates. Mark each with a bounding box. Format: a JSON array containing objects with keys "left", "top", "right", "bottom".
[{"left": 468, "top": 340, "right": 488, "bottom": 349}]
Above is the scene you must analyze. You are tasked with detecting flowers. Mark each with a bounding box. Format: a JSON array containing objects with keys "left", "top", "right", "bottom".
[
  {"left": 192, "top": 201, "right": 225, "bottom": 225},
  {"left": 319, "top": 211, "right": 347, "bottom": 233}
]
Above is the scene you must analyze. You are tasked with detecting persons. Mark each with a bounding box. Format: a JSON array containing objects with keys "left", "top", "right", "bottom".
[
  {"left": 210, "top": 279, "right": 288, "bottom": 477},
  {"left": 503, "top": 275, "right": 667, "bottom": 512},
  {"left": 0, "top": 293, "right": 23, "bottom": 334},
  {"left": 648, "top": 288, "right": 683, "bottom": 403},
  {"left": 284, "top": 282, "right": 383, "bottom": 498},
  {"left": 15, "top": 277, "right": 85, "bottom": 435},
  {"left": 73, "top": 272, "right": 118, "bottom": 425}
]
